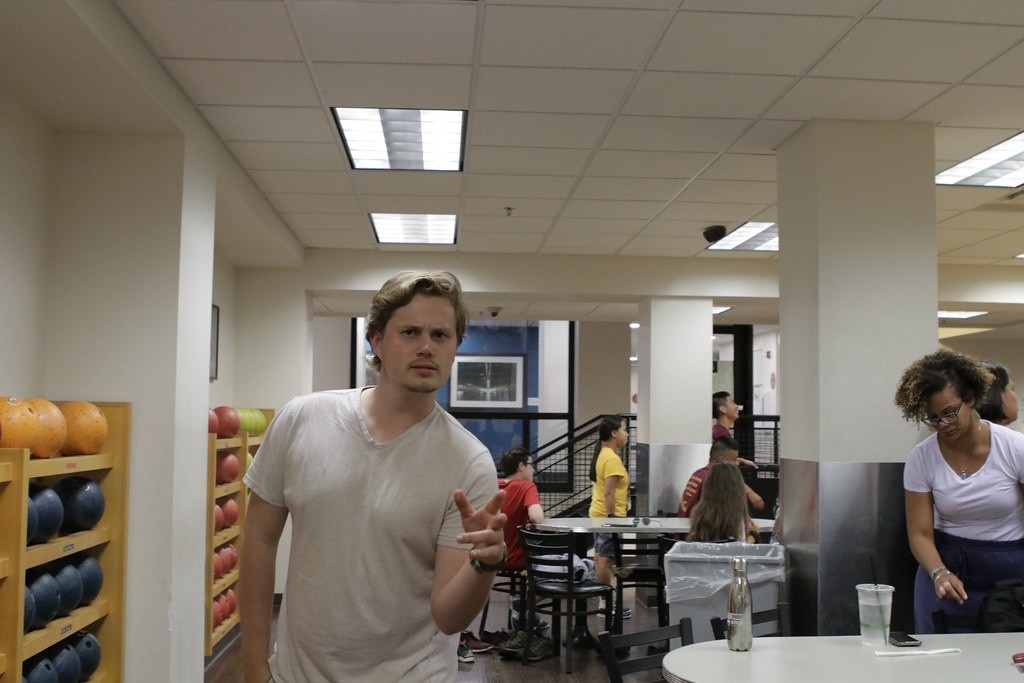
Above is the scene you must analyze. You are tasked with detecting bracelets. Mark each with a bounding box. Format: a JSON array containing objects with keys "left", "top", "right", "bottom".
[{"left": 931, "top": 566, "right": 951, "bottom": 584}]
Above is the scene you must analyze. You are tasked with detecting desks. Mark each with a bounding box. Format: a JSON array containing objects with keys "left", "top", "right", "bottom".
[
  {"left": 534, "top": 518, "right": 775, "bottom": 532},
  {"left": 663, "top": 633, "right": 1024, "bottom": 683}
]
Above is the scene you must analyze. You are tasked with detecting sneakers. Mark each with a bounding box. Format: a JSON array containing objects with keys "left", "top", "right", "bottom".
[
  {"left": 460, "top": 632, "right": 493, "bottom": 652},
  {"left": 598, "top": 599, "right": 632, "bottom": 619},
  {"left": 521, "top": 637, "right": 555, "bottom": 660},
  {"left": 458, "top": 640, "right": 475, "bottom": 662},
  {"left": 526, "top": 611, "right": 550, "bottom": 631},
  {"left": 500, "top": 631, "right": 537, "bottom": 653},
  {"left": 509, "top": 597, "right": 525, "bottom": 631}
]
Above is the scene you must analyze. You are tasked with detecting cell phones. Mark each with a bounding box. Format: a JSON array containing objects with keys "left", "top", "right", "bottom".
[{"left": 888, "top": 630, "right": 922, "bottom": 646}]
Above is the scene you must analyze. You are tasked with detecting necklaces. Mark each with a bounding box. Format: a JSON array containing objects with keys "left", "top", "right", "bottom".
[{"left": 943, "top": 426, "right": 981, "bottom": 481}]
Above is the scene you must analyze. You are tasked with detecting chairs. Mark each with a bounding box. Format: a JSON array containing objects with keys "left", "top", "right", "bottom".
[
  {"left": 931, "top": 609, "right": 979, "bottom": 634},
  {"left": 479, "top": 509, "right": 792, "bottom": 683}
]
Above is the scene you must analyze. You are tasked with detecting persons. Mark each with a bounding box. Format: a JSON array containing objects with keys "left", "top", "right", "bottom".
[
  {"left": 687, "top": 463, "right": 759, "bottom": 543},
  {"left": 895, "top": 348, "right": 1024, "bottom": 634},
  {"left": 498, "top": 448, "right": 550, "bottom": 633},
  {"left": 587, "top": 416, "right": 632, "bottom": 618},
  {"left": 236, "top": 269, "right": 508, "bottom": 683},
  {"left": 676, "top": 436, "right": 739, "bottom": 539},
  {"left": 712, "top": 392, "right": 766, "bottom": 510},
  {"left": 973, "top": 359, "right": 1019, "bottom": 426}
]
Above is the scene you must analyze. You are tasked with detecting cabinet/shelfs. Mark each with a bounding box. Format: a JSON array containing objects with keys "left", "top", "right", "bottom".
[
  {"left": 204, "top": 409, "right": 275, "bottom": 656},
  {"left": 0, "top": 402, "right": 131, "bottom": 683}
]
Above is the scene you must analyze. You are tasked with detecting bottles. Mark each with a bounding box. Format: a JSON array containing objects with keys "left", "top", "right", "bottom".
[{"left": 726, "top": 559, "right": 753, "bottom": 652}]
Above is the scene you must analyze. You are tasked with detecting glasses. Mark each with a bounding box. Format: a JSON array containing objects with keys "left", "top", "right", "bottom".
[
  {"left": 922, "top": 400, "right": 964, "bottom": 425},
  {"left": 524, "top": 462, "right": 537, "bottom": 469}
]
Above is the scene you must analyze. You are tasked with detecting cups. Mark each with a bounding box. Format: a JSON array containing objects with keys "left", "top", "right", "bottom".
[{"left": 855, "top": 583, "right": 895, "bottom": 646}]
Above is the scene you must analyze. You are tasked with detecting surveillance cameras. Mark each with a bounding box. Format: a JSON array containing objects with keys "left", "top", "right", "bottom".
[
  {"left": 486, "top": 307, "right": 502, "bottom": 317},
  {"left": 702, "top": 226, "right": 726, "bottom": 242}
]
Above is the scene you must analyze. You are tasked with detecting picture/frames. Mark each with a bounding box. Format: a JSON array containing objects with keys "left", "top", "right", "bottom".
[
  {"left": 448, "top": 353, "right": 528, "bottom": 413},
  {"left": 210, "top": 304, "right": 220, "bottom": 380}
]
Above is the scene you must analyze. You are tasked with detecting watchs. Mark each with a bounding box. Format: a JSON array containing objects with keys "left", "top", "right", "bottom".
[{"left": 469, "top": 541, "right": 507, "bottom": 572}]
